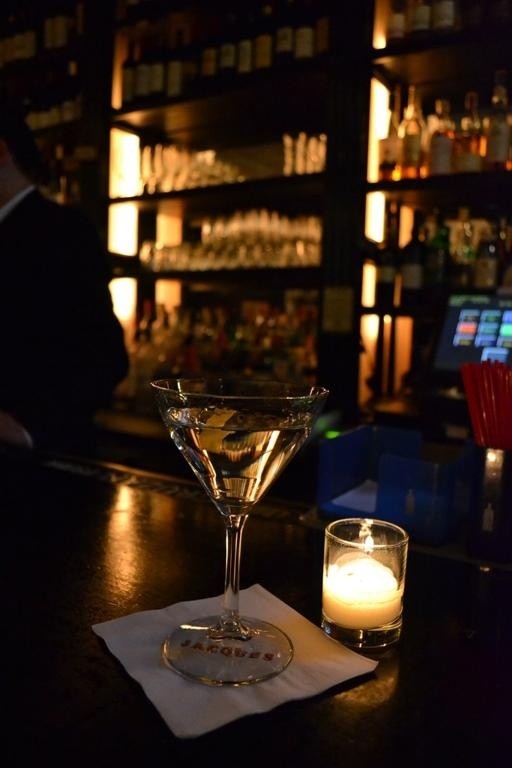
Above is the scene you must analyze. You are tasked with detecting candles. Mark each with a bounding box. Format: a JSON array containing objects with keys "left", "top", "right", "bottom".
[{"left": 321, "top": 517, "right": 409, "bottom": 631}]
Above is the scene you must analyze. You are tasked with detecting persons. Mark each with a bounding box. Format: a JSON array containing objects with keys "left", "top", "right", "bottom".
[{"left": 1, "top": 117, "right": 139, "bottom": 461}]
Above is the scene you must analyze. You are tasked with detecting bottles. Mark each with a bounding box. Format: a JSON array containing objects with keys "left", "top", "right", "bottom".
[
  {"left": 1, "top": 1, "right": 106, "bottom": 222},
  {"left": 113, "top": 2, "right": 367, "bottom": 384},
  {"left": 373, "top": 2, "right": 512, "bottom": 313}
]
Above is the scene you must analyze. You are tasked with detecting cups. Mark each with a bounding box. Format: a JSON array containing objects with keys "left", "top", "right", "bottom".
[{"left": 321, "top": 516, "right": 412, "bottom": 650}]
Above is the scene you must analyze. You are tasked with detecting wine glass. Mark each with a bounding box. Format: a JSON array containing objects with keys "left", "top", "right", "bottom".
[{"left": 151, "top": 374, "right": 332, "bottom": 689}]
